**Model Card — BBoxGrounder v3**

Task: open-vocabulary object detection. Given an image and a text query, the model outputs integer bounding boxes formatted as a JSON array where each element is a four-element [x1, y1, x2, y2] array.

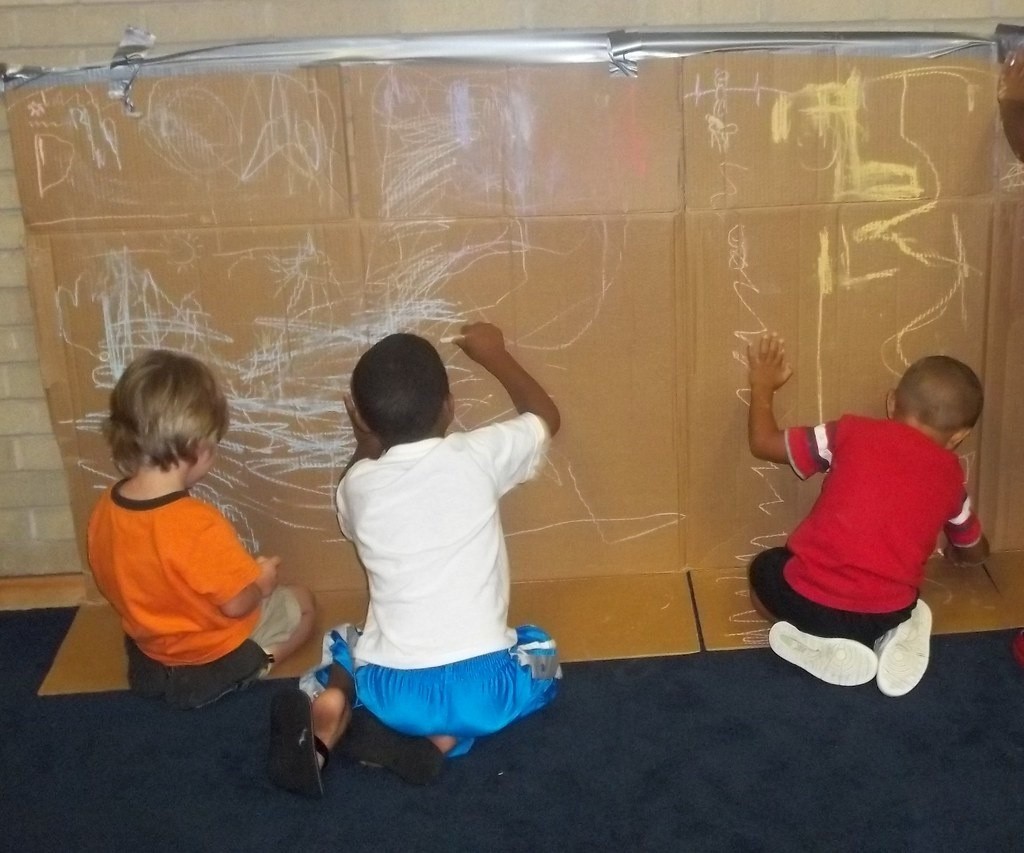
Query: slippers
[[349, 715, 445, 785], [269, 689, 326, 798]]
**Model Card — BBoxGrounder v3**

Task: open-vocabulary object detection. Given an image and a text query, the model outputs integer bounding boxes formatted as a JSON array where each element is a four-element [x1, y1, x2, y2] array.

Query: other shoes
[[872, 597, 933, 696], [123, 632, 168, 704], [768, 623, 878, 687], [169, 639, 268, 710]]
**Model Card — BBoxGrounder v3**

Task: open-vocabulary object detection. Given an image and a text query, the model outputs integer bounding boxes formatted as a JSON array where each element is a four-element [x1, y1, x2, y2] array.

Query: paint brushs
[[686, 571, 709, 654], [981, 563, 1002, 599]]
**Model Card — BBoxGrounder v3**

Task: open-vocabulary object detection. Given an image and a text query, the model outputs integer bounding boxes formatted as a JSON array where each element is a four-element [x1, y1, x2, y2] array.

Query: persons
[[264, 321, 562, 800], [86, 347, 315, 713], [746, 334, 991, 697]]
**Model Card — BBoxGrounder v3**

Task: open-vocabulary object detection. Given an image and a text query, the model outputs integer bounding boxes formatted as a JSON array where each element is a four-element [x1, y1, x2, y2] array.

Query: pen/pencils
[[440, 335, 465, 343]]
